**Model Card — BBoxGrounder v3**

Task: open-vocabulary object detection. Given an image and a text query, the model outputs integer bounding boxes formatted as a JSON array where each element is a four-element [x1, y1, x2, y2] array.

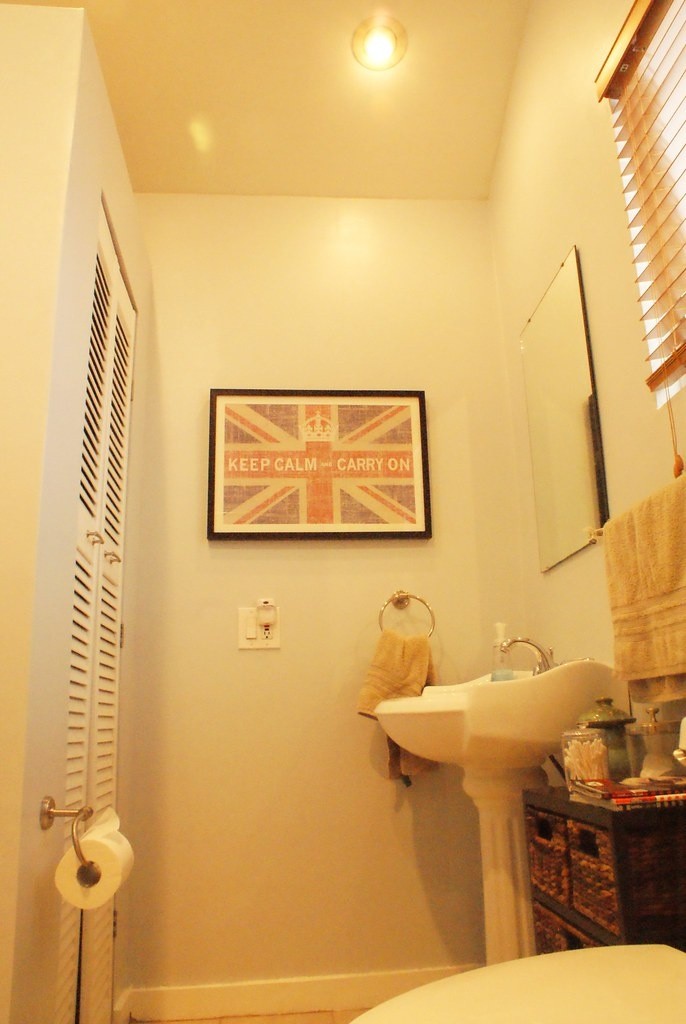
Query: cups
[[562, 696, 685, 796]]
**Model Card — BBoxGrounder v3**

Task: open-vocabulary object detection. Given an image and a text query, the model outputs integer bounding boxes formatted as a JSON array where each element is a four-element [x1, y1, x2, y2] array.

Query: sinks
[[376, 657, 616, 770]]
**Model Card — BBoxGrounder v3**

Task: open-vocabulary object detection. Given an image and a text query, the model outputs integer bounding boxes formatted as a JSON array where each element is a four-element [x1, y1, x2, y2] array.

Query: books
[[569, 777, 686, 811]]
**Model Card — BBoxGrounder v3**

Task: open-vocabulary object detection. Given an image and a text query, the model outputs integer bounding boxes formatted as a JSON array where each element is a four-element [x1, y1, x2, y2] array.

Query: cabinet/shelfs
[[522, 779, 686, 956]]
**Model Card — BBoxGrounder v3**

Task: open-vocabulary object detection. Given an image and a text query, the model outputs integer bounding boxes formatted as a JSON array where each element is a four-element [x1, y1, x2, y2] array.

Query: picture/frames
[[208, 389, 433, 539]]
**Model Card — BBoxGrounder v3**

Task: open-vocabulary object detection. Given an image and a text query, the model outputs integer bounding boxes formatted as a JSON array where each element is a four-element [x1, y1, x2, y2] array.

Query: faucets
[[500, 637, 555, 678]]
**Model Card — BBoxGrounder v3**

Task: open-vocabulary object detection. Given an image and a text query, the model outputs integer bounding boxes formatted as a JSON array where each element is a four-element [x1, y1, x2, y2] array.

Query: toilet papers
[[54, 807, 133, 912]]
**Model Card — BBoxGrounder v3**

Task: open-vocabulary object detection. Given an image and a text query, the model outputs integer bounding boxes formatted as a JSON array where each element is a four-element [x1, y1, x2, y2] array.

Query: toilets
[[349, 943, 685, 1024]]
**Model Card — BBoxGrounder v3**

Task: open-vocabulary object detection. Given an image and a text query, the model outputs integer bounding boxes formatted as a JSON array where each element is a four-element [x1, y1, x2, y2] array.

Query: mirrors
[[517, 244, 609, 573]]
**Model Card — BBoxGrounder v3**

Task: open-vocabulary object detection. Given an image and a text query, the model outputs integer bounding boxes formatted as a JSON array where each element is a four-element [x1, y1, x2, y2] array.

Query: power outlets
[[238, 607, 280, 650]]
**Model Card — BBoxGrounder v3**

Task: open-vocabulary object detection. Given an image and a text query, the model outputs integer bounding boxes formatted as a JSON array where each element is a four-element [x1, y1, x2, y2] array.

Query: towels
[[355, 629, 439, 788]]
